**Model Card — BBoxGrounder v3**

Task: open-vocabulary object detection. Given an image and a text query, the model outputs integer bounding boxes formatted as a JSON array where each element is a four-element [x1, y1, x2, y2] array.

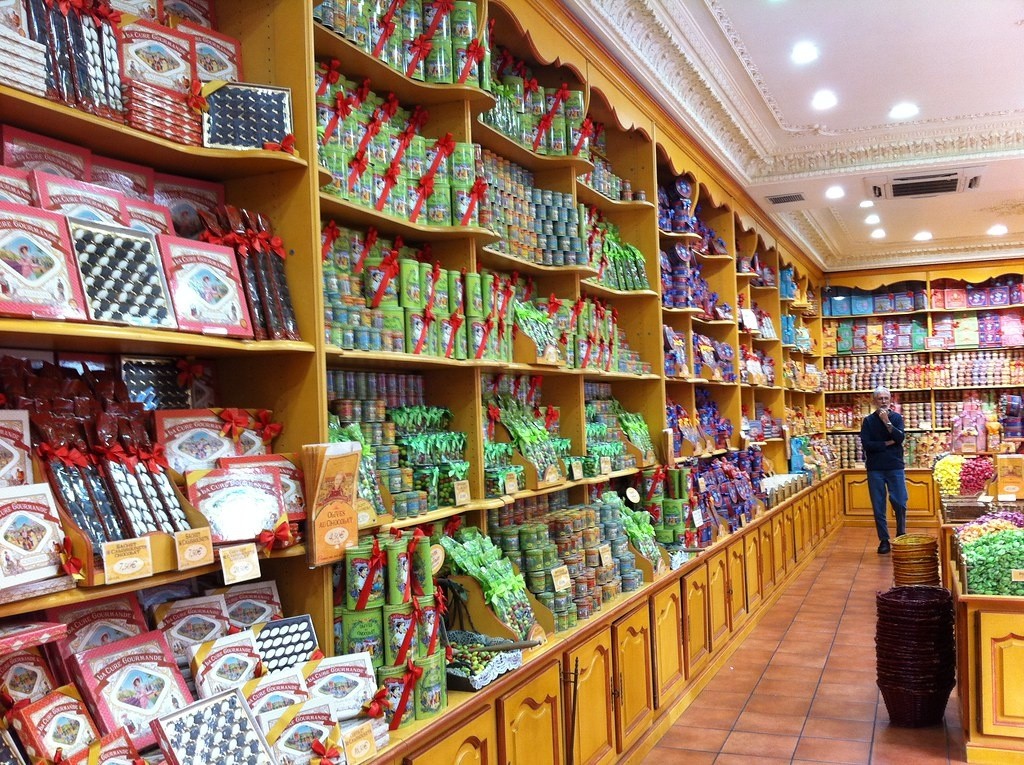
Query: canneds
[[824, 350, 1024, 469], [311, 0, 691, 727]]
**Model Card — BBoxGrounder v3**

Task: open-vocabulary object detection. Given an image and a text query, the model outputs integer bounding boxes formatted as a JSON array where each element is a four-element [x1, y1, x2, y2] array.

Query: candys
[[933, 455, 1024, 597]]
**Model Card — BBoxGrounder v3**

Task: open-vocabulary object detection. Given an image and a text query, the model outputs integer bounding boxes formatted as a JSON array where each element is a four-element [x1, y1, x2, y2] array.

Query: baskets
[[875, 585, 957, 728], [887, 533, 941, 587]]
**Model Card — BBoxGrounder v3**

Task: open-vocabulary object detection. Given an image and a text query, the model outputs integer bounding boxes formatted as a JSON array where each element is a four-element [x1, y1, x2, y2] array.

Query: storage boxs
[[1, 581, 387, 765], [1, 121, 253, 339], [820, 284, 1024, 352], [0, 0, 294, 151], [0, 344, 313, 589], [742, 400, 782, 443]]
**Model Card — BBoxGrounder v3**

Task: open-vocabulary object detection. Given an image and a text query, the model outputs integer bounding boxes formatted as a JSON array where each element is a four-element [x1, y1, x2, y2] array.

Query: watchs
[[886, 422, 892, 428]]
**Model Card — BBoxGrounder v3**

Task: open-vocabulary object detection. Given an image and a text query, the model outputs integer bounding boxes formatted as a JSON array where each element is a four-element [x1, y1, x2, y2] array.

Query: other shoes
[[878, 541, 890, 553]]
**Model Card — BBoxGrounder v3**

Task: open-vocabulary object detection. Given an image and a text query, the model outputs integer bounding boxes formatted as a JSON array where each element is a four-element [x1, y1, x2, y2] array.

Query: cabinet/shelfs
[[0, 0, 1024, 765]]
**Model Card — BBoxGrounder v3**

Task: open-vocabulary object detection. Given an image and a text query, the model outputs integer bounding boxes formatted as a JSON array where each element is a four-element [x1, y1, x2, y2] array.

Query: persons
[[861, 385, 909, 554]]
